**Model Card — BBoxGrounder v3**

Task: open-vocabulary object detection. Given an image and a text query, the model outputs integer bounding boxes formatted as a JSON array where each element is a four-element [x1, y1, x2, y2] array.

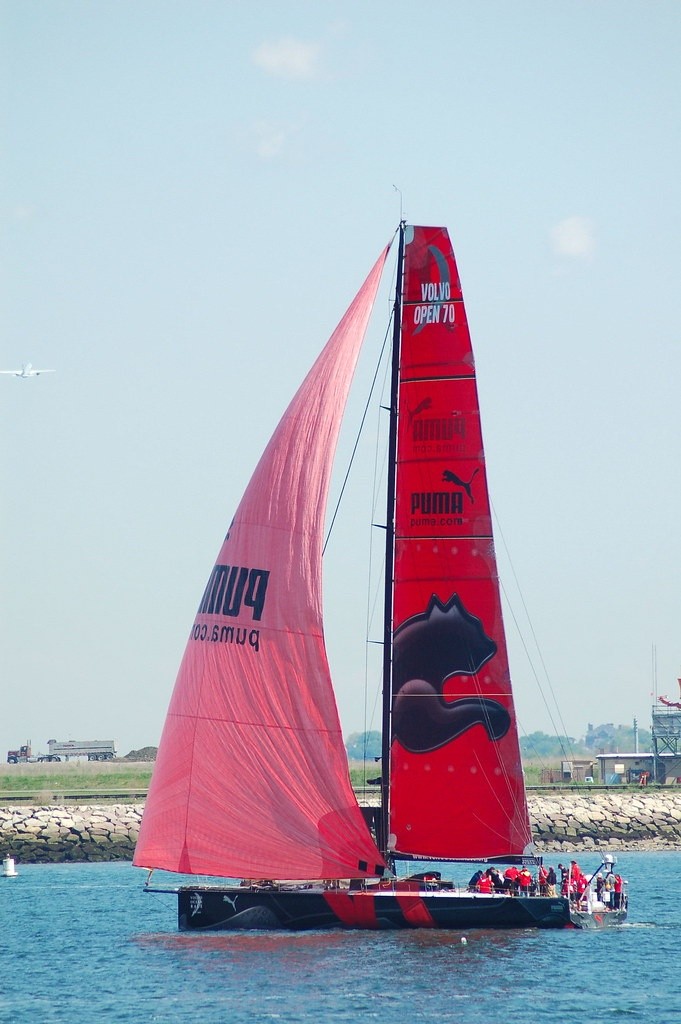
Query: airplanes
[[1, 360, 53, 380]]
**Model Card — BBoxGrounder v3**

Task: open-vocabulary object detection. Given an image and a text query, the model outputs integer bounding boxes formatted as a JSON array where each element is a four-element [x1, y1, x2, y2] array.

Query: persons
[[468, 861, 621, 910]]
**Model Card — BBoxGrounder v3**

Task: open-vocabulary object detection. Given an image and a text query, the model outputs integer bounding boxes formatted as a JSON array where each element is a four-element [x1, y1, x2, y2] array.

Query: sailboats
[[130, 184, 583, 934]]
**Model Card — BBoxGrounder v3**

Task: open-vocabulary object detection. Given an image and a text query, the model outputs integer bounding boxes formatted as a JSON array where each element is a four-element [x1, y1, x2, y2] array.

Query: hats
[[570, 860, 577, 864], [549, 866, 554, 872], [597, 873, 602, 878], [603, 869, 611, 873], [521, 868, 527, 872], [512, 866, 517, 869]]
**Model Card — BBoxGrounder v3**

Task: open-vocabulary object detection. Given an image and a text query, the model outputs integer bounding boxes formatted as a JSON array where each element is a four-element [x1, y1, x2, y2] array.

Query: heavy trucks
[[7, 739, 117, 764]]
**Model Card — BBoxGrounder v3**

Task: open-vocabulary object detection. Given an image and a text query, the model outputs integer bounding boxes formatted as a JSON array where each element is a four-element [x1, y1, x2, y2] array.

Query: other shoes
[[577, 906, 581, 910], [606, 909, 611, 912]]
